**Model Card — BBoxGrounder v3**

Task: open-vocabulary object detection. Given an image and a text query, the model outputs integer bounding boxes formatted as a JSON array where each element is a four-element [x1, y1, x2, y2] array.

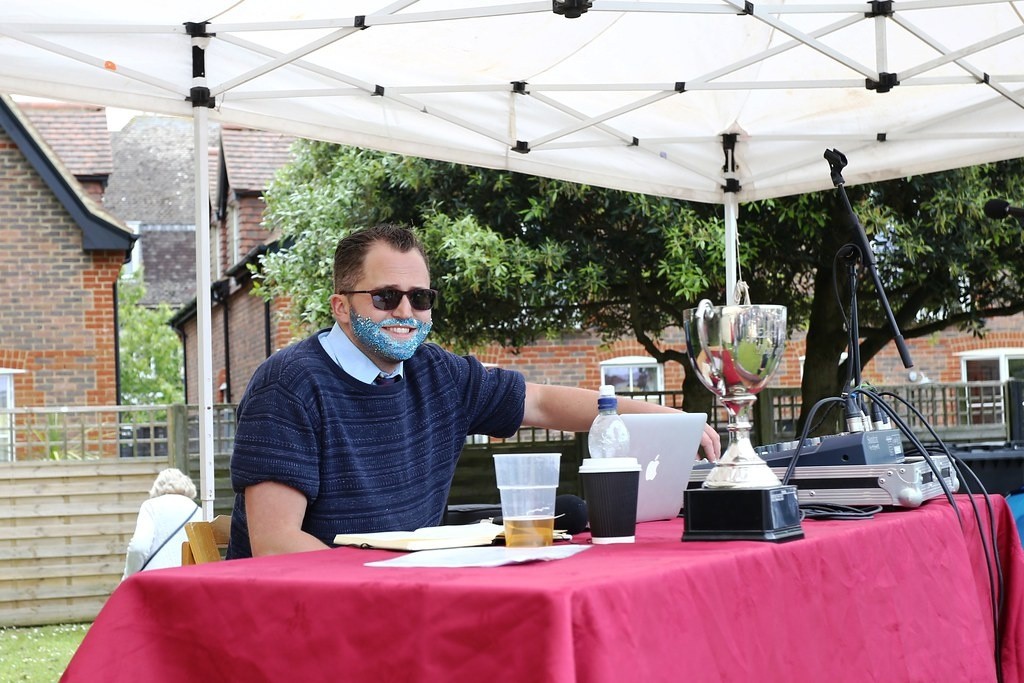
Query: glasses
[[340, 288, 438, 310]]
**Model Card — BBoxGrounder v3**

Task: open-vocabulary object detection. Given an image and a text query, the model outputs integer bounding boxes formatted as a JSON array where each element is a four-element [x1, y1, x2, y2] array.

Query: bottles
[[588, 385, 630, 458]]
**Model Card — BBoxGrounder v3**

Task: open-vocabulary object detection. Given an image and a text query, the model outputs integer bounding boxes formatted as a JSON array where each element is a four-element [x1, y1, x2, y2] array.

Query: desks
[[58, 493, 1024, 683]]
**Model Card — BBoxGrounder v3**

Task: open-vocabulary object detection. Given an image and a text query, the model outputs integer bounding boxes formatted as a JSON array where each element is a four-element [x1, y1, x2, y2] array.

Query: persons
[[224, 225, 721, 561], [121, 469, 203, 582]]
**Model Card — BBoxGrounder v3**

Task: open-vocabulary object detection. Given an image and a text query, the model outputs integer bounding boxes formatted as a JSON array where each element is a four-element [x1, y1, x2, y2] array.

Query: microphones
[[470, 496, 590, 536]]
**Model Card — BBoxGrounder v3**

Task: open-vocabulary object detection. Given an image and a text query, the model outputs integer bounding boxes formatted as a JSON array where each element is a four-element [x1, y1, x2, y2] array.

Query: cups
[[493, 453, 562, 548], [579, 458, 643, 545]]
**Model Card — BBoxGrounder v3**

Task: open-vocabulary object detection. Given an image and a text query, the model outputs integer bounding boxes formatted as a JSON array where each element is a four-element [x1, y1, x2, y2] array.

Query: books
[[334, 523, 571, 551]]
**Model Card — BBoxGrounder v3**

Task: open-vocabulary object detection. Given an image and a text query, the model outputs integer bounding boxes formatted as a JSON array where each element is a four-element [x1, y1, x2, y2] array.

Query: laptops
[[619, 411, 708, 523]]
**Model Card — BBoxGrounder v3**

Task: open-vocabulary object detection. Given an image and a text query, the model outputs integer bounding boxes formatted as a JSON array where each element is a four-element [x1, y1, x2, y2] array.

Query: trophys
[[682, 299, 804, 542]]
